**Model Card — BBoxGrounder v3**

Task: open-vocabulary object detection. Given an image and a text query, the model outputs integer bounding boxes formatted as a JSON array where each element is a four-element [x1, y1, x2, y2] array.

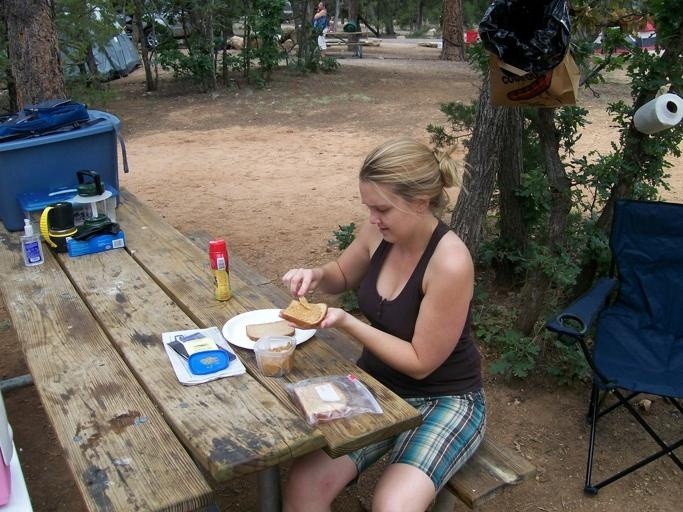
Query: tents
[[592, 15, 663, 54], [55, 3, 144, 82]]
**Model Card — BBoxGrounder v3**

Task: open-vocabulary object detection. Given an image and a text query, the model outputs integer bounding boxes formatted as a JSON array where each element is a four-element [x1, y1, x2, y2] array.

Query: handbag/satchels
[[0, 99, 90, 142], [478, 0, 580, 107]]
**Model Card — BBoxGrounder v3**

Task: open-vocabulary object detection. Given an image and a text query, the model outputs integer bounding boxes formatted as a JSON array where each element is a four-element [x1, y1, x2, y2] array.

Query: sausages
[[507, 72, 552, 101]]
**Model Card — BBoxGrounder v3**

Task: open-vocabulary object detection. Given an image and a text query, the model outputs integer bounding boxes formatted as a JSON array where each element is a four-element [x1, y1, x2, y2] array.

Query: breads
[[283, 299, 327, 328], [246, 319, 295, 340], [292, 382, 348, 424]]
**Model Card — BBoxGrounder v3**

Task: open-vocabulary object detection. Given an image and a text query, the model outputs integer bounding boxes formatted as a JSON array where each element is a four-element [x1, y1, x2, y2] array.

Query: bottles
[[208, 239, 232, 301], [21, 218, 44, 267]]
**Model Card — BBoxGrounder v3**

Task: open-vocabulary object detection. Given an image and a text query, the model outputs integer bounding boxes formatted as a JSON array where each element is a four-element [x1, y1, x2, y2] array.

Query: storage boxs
[[0, 110, 120, 231]]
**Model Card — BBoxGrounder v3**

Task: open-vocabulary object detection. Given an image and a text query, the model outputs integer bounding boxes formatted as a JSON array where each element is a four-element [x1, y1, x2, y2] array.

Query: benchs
[[327, 43, 369, 58], [173, 224, 535, 512]]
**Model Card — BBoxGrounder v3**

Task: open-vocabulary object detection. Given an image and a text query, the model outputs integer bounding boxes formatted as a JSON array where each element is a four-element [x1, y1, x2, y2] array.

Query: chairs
[[544, 197, 683, 495]]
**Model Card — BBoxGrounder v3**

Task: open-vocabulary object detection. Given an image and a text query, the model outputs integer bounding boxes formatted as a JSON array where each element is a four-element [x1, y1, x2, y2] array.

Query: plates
[[221, 309, 318, 351]]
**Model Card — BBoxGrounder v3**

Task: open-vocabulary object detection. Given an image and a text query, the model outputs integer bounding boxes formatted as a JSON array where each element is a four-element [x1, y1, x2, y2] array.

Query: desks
[[0, 197, 420, 512], [324, 32, 367, 58]]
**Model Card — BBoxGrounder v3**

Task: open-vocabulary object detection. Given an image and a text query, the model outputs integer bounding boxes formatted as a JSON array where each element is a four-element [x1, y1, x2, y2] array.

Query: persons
[[279, 139, 486, 512], [312, 1, 330, 57]]
